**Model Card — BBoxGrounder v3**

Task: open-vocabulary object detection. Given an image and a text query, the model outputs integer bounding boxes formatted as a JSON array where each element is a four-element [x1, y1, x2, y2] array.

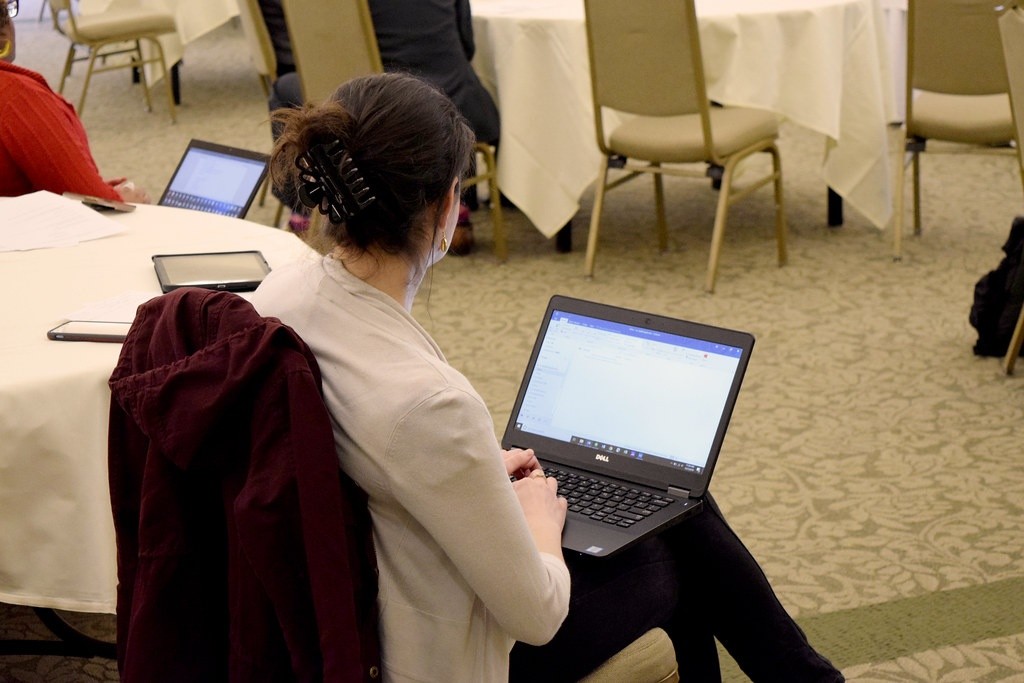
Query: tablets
[[151, 250, 273, 295]]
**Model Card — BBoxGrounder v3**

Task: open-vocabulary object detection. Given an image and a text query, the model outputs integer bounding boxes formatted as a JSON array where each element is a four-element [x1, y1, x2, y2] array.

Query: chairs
[[281, 0, 508, 265], [108, 287, 680, 683], [584, 0, 789, 295], [48, 0, 178, 127], [237, 0, 278, 101], [893, 0, 1024, 261]]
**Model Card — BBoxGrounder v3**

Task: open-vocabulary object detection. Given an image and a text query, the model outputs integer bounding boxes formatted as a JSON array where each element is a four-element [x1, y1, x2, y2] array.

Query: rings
[[534, 474, 549, 484]]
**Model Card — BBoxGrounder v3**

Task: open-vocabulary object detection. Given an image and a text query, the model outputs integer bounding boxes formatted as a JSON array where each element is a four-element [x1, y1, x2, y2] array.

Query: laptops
[[156, 139, 272, 219], [500, 295, 756, 562]]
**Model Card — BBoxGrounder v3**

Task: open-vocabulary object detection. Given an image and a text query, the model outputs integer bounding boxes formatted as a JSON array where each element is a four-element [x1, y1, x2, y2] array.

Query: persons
[[249, 70, 844, 683], [0, 1, 154, 206], [261, 0, 297, 83], [269, 2, 501, 257]]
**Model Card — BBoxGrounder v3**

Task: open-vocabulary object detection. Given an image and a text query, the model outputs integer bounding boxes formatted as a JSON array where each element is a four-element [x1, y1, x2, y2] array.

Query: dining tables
[[78, 0, 240, 107], [0, 196, 330, 661], [470, 1, 909, 256]]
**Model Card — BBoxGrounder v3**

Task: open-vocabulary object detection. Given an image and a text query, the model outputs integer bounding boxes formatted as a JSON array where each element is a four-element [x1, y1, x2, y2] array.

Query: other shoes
[[446, 225, 476, 257]]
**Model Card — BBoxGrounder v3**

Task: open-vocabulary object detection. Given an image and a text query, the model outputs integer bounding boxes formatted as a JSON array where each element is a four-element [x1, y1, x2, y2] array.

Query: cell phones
[[62, 191, 136, 212], [47, 319, 133, 343]]
